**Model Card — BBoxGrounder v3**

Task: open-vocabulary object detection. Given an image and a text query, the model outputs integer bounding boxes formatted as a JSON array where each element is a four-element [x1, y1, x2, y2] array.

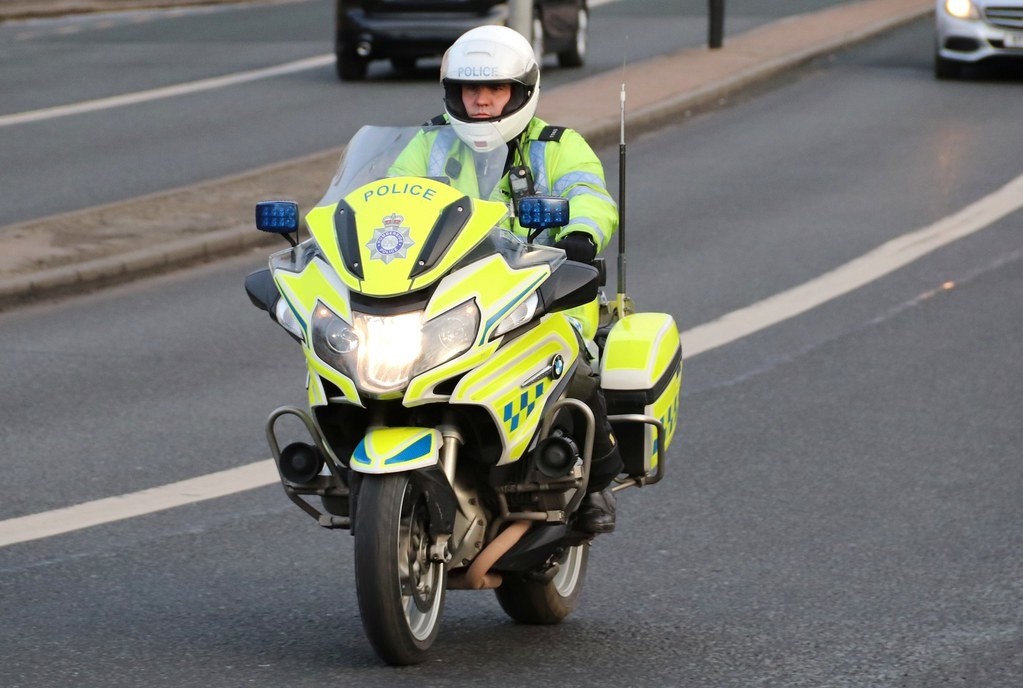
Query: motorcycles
[[242, 125, 687, 667]]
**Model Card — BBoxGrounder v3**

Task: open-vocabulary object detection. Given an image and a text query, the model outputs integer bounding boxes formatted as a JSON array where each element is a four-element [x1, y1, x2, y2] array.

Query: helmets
[[440, 25, 539, 153]]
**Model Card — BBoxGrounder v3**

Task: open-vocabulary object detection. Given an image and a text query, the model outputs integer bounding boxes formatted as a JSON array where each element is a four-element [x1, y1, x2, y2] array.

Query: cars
[[333, 1, 592, 80], [933, 1, 1023, 80]]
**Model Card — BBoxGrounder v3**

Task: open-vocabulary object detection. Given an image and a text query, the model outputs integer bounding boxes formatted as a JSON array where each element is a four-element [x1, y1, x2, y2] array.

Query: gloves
[[552, 231, 597, 264], [290, 242, 319, 265]]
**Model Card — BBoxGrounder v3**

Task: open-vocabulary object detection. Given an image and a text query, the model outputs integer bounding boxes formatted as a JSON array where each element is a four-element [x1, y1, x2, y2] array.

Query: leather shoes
[[579, 484, 618, 533]]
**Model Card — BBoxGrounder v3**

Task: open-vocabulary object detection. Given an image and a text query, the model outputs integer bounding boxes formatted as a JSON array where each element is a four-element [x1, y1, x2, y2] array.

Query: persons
[[383, 24, 625, 533]]
